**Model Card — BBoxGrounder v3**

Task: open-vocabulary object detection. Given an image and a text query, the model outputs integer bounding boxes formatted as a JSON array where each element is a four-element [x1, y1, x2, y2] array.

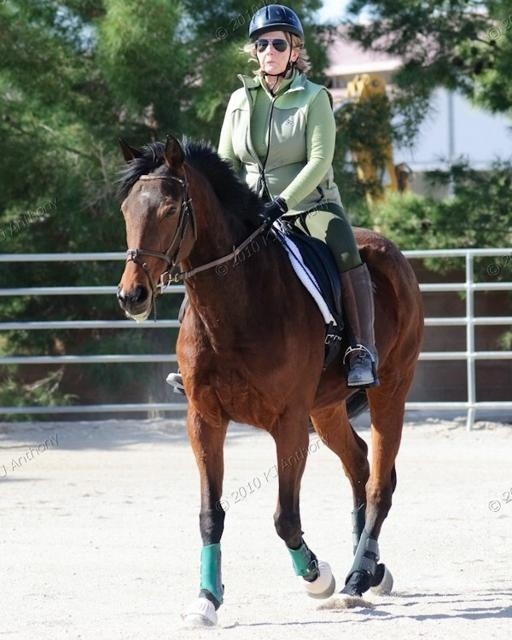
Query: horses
[[113, 131, 426, 627]]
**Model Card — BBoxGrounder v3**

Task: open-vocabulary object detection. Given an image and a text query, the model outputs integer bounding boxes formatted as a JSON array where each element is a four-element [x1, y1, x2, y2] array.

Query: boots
[[166, 371, 185, 391], [334, 259, 379, 388]]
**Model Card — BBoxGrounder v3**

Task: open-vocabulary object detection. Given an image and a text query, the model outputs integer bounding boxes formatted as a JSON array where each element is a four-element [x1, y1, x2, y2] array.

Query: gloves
[[256, 197, 291, 232]]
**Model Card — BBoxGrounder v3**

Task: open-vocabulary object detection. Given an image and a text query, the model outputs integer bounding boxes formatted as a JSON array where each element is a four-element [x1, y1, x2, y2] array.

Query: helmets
[[246, 4, 305, 39]]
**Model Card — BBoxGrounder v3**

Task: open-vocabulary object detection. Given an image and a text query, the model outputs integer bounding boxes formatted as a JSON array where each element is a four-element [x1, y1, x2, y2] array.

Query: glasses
[[254, 39, 289, 53]]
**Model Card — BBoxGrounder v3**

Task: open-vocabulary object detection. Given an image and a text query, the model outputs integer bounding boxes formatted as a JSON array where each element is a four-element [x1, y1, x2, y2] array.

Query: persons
[[164, 3, 380, 392]]
